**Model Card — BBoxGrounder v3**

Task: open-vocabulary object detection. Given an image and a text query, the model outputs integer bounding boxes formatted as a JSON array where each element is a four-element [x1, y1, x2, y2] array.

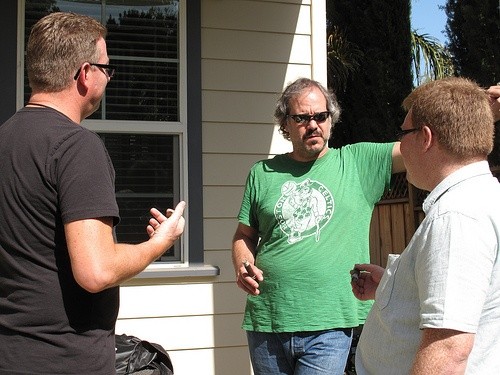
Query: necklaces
[[27, 101, 72, 120]]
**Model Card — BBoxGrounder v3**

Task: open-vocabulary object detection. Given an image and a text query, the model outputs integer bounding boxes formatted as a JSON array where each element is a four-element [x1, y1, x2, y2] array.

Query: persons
[[348, 75, 500, 375], [0, 10, 186, 375], [231, 77, 500, 375]]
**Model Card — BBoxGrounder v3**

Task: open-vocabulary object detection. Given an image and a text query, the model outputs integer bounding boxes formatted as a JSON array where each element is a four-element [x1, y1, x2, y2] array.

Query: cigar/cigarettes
[[352, 264, 360, 282], [243, 260, 259, 284]]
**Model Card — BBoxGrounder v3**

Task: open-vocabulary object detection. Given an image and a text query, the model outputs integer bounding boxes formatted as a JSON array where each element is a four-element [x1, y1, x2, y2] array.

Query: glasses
[[394, 127, 434, 142], [74, 64, 116, 80], [285, 111, 330, 123]]
